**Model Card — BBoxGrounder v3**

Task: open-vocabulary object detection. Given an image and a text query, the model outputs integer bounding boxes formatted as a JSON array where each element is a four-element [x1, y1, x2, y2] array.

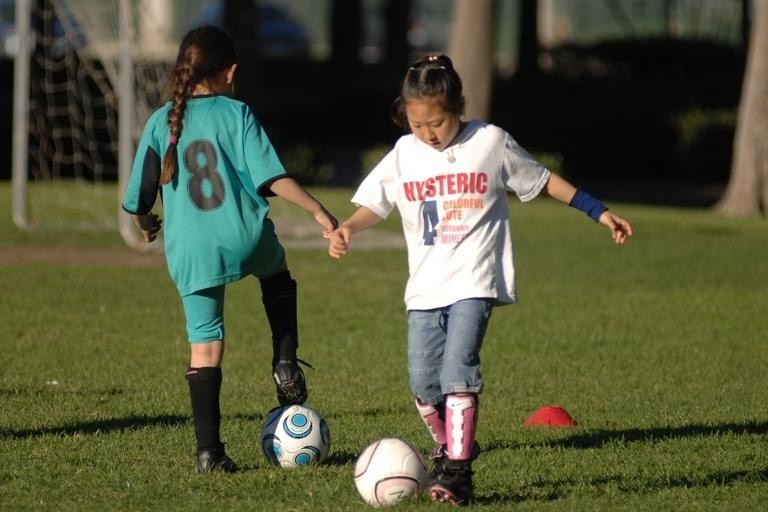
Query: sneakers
[[195, 448, 235, 471], [274, 357, 315, 403], [427, 438, 481, 506]]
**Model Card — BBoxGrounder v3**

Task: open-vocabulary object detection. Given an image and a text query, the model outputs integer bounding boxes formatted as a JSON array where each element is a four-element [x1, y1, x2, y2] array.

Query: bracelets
[[567, 187, 610, 224]]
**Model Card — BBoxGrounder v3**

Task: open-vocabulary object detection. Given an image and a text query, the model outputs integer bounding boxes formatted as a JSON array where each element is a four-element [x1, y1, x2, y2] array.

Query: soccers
[[260, 404, 330, 466], [354, 437, 429, 509]]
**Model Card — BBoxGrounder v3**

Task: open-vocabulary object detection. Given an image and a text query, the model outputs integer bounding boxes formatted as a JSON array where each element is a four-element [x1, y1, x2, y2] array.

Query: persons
[[121, 25, 339, 475], [327, 54, 634, 508]]
